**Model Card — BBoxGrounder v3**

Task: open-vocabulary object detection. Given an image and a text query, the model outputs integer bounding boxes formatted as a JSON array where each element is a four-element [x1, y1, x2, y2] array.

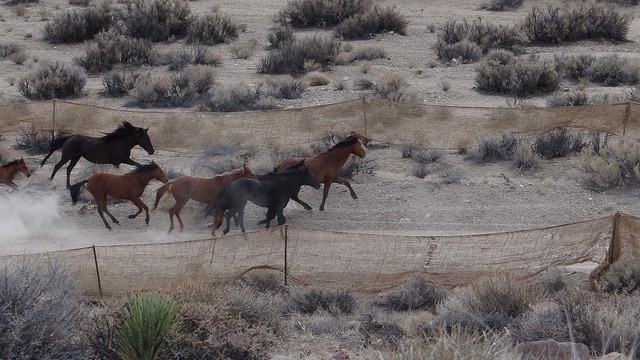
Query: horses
[[41, 120, 154, 189], [151, 162, 256, 237], [201, 165, 321, 235], [0, 158, 30, 194], [232, 159, 305, 227], [274, 131, 366, 211], [70, 159, 168, 231]]
